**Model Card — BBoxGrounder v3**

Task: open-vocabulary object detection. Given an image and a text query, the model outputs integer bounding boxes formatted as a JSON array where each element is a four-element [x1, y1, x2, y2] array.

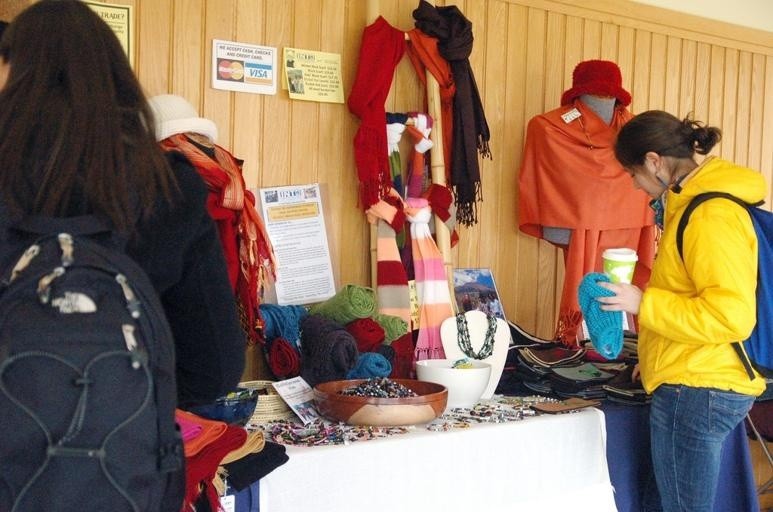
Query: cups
[[602, 248, 639, 284]]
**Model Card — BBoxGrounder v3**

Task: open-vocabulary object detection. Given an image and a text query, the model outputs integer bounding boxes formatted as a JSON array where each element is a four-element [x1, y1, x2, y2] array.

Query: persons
[[1, 0, 187, 512], [519, 59, 658, 347], [593, 110, 766, 512], [145, 94, 277, 352]]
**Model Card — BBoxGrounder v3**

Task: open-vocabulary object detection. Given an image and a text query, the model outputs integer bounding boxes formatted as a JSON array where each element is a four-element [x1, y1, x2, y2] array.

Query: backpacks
[[677, 192, 773, 379], [0, 212, 185, 512]]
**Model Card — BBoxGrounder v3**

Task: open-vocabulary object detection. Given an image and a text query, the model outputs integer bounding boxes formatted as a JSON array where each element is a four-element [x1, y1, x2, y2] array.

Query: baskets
[[238, 380, 295, 423]]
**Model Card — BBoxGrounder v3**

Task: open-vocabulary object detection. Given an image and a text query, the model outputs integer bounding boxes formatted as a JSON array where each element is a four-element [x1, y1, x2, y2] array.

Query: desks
[[195, 365, 759, 512]]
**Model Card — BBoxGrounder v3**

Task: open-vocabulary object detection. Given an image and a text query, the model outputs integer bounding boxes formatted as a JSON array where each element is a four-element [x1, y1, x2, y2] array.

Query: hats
[[561, 61, 631, 106], [578, 272, 623, 359], [138, 94, 219, 143]]
